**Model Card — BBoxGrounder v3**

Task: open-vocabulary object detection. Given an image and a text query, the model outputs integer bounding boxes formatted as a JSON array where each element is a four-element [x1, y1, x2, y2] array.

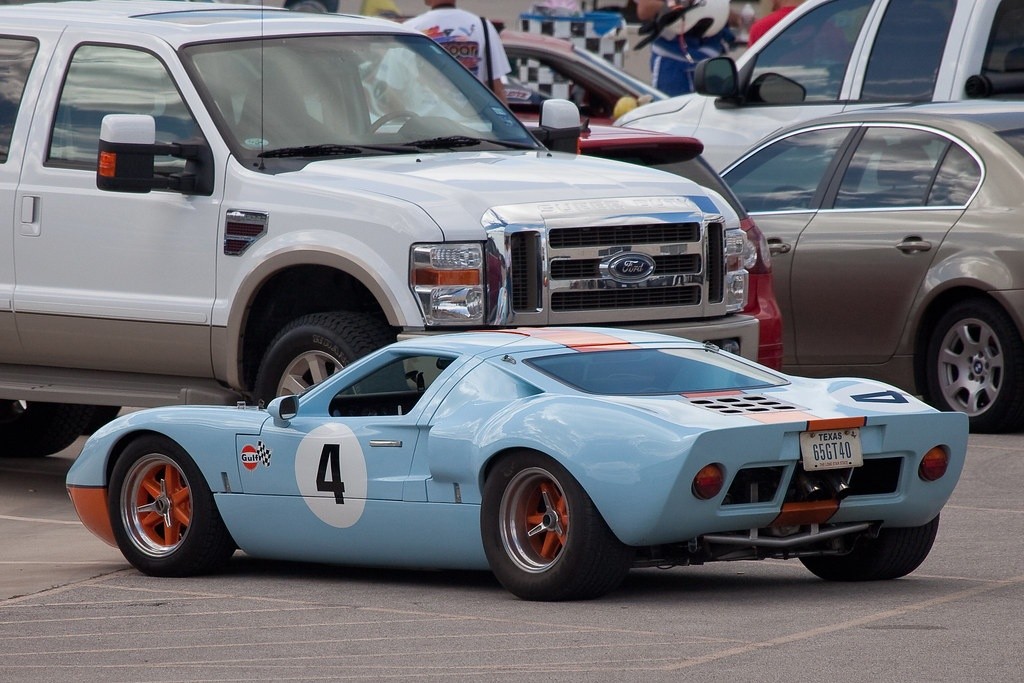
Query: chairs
[[873, 4, 946, 79], [74, 67, 177, 152], [238, 78, 333, 147], [873, 144, 932, 205]]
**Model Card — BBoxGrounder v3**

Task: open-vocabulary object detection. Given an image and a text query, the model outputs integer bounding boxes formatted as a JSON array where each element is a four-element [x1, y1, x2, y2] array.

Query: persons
[[376, 0, 512, 131], [634, 0, 847, 97]]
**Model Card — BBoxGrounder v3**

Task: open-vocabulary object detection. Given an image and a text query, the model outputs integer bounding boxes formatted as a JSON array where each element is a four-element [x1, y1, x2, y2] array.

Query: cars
[[715, 109, 1024, 433]]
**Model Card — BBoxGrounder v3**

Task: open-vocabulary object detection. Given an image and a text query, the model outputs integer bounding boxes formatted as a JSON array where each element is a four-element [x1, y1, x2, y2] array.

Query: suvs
[[0, 0, 762, 457], [359, 0, 1024, 375]]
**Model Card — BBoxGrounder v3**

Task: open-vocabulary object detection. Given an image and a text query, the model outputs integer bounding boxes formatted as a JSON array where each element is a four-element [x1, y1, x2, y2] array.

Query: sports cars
[[63, 322, 972, 600]]
